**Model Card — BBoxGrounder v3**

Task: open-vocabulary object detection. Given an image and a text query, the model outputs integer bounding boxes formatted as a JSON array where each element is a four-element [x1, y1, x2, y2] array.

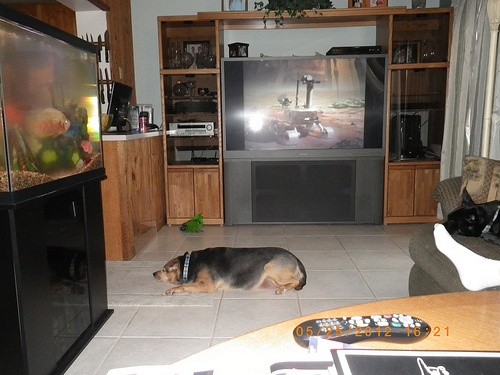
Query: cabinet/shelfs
[[165, 166, 224, 228], [156, 6, 455, 166], [385, 163, 438, 225]]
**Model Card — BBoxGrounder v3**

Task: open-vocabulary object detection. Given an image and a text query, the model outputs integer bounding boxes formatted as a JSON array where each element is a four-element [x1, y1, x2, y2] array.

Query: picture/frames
[[348, 0, 388, 8], [330, 348, 500, 375], [222, 0, 249, 11], [392, 40, 420, 63], [183, 40, 210, 69]]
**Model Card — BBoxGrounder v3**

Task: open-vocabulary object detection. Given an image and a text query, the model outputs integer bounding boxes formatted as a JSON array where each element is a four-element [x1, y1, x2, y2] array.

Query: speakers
[[391, 114, 422, 159]]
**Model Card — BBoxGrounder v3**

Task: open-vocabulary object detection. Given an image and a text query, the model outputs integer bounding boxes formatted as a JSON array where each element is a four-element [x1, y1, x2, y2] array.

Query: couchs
[[408, 176, 499, 297]]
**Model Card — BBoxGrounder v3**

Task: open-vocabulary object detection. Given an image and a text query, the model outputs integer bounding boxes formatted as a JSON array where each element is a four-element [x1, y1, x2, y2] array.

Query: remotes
[[293, 312, 432, 349]]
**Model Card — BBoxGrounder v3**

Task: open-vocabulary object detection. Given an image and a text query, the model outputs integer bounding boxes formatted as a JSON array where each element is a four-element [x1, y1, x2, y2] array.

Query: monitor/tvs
[[221, 54, 388, 159], [107, 81, 132, 134]]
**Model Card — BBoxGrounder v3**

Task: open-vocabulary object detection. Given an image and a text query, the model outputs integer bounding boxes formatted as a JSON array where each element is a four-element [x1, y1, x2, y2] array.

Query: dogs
[[153, 247, 306, 295], [433, 187, 500, 237]]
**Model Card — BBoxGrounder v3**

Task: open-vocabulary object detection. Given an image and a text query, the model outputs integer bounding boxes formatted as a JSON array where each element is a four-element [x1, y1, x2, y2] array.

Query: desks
[[170, 291, 500, 375], [101, 128, 165, 263]]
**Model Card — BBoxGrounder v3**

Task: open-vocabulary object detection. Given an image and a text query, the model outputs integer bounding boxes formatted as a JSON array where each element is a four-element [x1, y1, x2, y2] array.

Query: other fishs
[[24, 108, 71, 139]]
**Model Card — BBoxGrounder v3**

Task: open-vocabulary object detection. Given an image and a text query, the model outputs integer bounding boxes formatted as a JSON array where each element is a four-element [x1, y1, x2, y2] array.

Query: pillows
[[458, 152, 500, 204]]
